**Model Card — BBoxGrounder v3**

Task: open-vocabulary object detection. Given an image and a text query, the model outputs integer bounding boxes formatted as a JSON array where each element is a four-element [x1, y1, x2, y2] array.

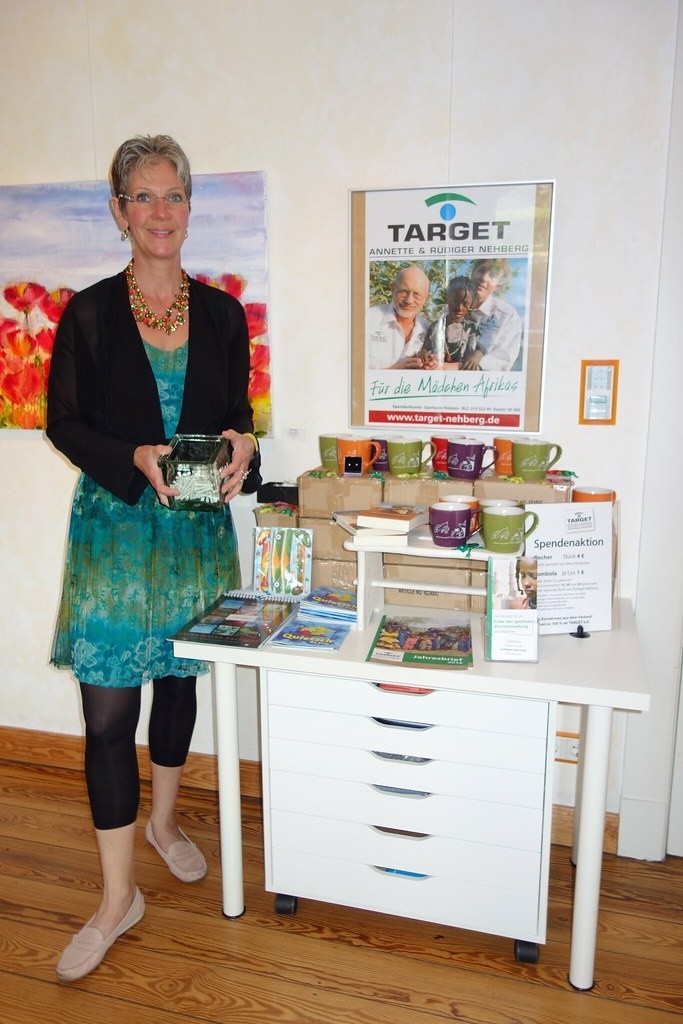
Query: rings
[[240, 469, 252, 481]]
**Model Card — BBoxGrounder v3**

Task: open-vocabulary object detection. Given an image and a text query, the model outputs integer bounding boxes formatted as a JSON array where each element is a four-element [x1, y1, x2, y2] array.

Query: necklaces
[[125, 261, 190, 334]]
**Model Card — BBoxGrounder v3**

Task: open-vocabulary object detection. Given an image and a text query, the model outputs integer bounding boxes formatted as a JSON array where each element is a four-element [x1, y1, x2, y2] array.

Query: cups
[[430, 435, 465, 473], [319, 434, 353, 471], [512, 439, 562, 480], [370, 434, 401, 470], [478, 498, 525, 539], [440, 493, 479, 532], [337, 436, 382, 476], [483, 507, 537, 551], [446, 439, 499, 480], [572, 486, 616, 509], [429, 502, 483, 547], [387, 437, 436, 476], [494, 436, 528, 475]]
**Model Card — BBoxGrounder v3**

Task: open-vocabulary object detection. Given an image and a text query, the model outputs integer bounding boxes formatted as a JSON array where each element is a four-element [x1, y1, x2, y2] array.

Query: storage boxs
[[158, 433, 234, 509], [256, 462, 572, 611]]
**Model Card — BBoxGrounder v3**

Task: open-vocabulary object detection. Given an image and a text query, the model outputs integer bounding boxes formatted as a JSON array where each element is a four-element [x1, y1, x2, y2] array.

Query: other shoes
[[56, 887, 146, 982], [146, 819, 208, 882]]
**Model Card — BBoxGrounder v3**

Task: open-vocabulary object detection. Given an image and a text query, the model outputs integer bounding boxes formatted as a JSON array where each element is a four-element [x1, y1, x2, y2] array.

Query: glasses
[[118, 193, 190, 208]]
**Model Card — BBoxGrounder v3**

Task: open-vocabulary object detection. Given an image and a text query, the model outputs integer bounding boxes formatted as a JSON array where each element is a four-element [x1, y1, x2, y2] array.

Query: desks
[[173, 599, 653, 991]]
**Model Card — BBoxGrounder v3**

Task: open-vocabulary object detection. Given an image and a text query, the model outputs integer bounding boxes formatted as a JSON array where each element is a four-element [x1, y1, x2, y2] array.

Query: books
[[166, 528, 358, 652]]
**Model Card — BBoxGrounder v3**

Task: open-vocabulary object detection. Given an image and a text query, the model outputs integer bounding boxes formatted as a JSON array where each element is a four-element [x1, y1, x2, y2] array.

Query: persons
[[419, 277, 479, 370], [506, 559, 537, 610], [380, 620, 472, 653], [425, 259, 523, 371], [45, 138, 259, 980], [369, 268, 437, 370]]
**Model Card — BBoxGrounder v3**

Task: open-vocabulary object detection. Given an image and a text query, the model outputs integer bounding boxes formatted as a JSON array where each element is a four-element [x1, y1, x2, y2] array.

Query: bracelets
[[242, 433, 258, 456]]
